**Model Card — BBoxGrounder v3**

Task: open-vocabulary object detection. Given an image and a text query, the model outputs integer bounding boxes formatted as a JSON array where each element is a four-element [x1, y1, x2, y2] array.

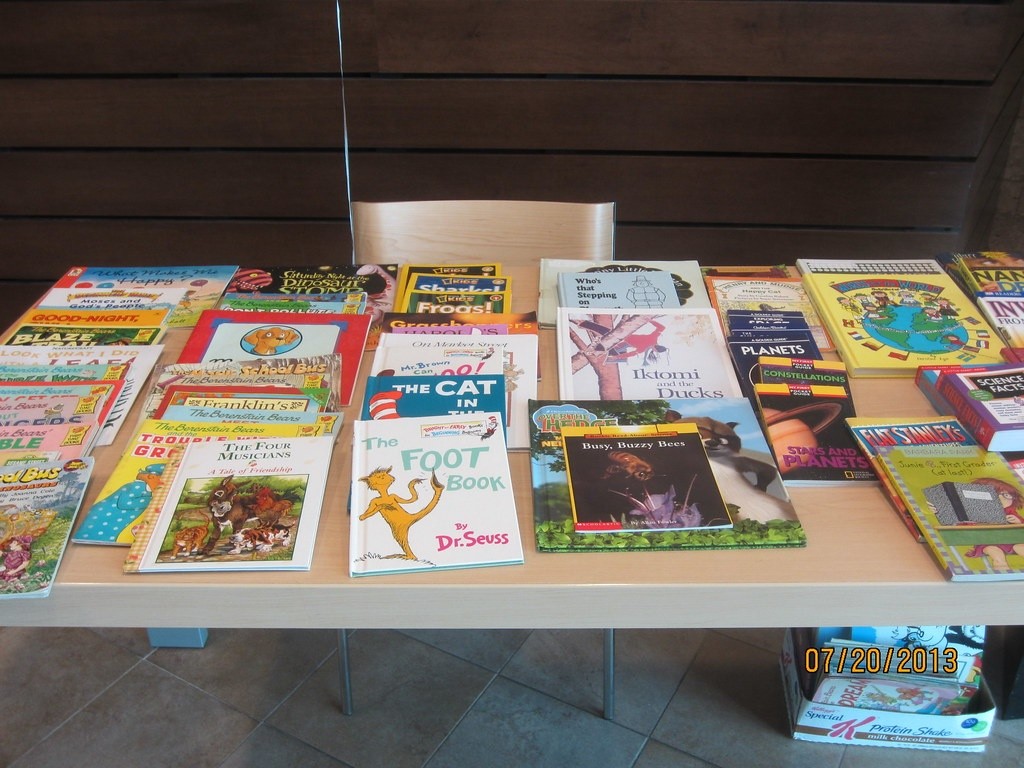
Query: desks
[[0, 265, 1024, 629]]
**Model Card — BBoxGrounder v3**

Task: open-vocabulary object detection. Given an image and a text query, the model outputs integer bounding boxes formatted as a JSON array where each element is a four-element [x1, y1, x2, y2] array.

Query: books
[[528, 396, 807, 552], [376, 260, 542, 381], [345, 373, 508, 514], [0, 262, 398, 600], [555, 269, 681, 309], [555, 305, 743, 399], [561, 422, 733, 534], [353, 331, 541, 452], [790, 624, 987, 718], [700, 252, 1023, 582], [351, 410, 524, 578], [536, 257, 712, 331]]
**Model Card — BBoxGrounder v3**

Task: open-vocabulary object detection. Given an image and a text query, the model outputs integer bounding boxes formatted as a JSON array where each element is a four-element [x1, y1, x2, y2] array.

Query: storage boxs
[[778, 625, 997, 753]]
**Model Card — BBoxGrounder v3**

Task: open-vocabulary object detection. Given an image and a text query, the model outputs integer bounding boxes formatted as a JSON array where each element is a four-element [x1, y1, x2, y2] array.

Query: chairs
[[336, 200, 618, 720]]
